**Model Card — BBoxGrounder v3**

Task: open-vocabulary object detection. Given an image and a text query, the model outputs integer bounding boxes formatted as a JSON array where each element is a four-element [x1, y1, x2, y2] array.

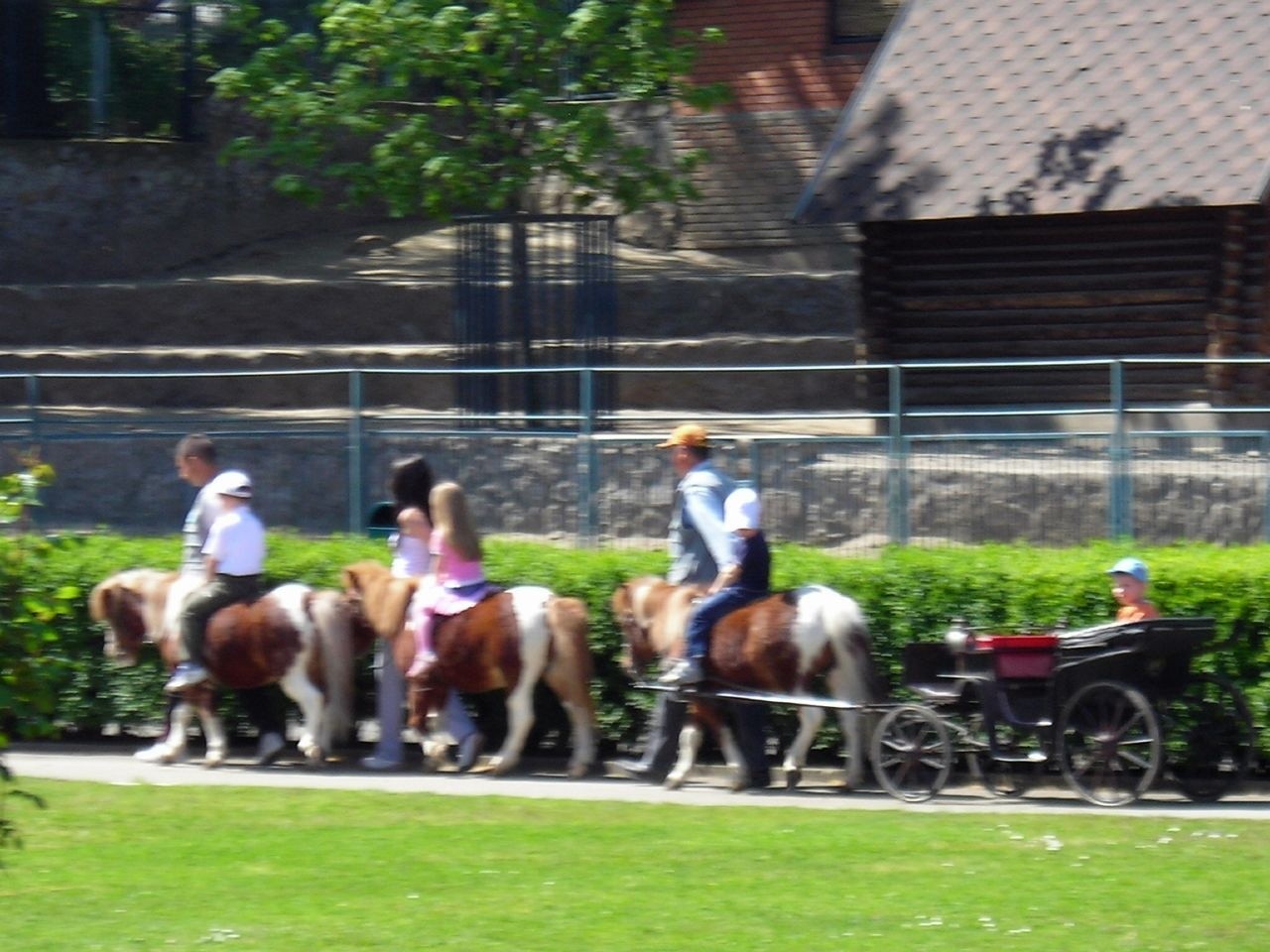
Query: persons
[[361, 454, 486, 774], [1104, 556, 1158, 623], [624, 424, 772, 792], [132, 432, 286, 763]]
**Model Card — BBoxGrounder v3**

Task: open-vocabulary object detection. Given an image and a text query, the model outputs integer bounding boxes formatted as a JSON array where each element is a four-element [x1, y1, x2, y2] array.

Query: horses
[[610, 573, 884, 796], [340, 560, 602, 779], [88, 569, 378, 771]]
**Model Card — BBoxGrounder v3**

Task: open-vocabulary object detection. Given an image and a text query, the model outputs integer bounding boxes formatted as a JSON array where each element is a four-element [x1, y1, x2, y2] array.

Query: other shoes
[[659, 662, 705, 686], [404, 655, 439, 679], [359, 756, 403, 770], [163, 661, 207, 691], [456, 733, 485, 771], [749, 768, 771, 787], [255, 729, 286, 763], [622, 764, 667, 783]]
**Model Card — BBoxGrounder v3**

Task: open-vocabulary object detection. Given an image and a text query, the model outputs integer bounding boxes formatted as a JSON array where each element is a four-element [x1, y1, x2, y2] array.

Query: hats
[[211, 471, 252, 498], [1101, 557, 1149, 583], [654, 425, 707, 449], [722, 489, 759, 533]]
